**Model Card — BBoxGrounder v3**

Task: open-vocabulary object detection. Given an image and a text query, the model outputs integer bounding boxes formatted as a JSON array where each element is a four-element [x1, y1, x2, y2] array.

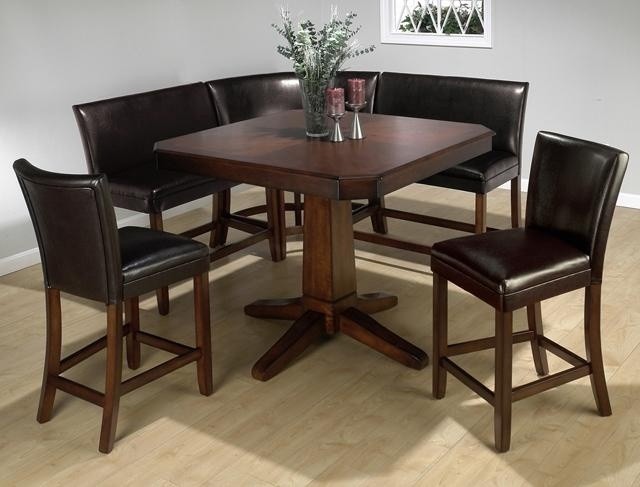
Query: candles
[[347, 78, 365, 105], [325, 86, 345, 114]]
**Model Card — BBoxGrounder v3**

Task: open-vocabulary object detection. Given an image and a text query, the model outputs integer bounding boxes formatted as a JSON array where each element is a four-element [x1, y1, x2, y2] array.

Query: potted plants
[[271, 2, 375, 137]]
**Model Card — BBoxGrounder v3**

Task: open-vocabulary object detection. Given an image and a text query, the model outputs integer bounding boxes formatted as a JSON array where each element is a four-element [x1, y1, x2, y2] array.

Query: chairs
[[72, 81, 281, 316], [431, 130, 628, 453], [205, 71, 380, 260], [13, 158, 214, 453], [352, 72, 529, 258]]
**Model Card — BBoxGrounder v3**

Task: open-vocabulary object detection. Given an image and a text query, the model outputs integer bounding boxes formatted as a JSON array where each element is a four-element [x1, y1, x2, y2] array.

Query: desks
[[155, 109, 497, 382]]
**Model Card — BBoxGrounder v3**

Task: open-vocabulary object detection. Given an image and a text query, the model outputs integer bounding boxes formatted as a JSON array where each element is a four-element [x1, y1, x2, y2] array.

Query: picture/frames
[[379, 0, 492, 48]]
